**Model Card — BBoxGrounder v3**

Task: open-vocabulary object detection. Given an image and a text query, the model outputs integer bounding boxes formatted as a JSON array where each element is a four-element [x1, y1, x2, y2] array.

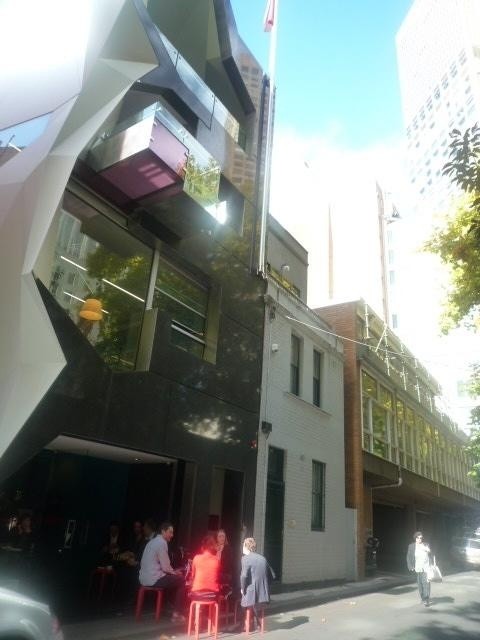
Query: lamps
[[79, 299, 103, 321]]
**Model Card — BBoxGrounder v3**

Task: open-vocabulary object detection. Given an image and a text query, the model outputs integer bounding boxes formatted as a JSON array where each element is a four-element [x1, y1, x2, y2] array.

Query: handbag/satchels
[[426, 555, 445, 585]]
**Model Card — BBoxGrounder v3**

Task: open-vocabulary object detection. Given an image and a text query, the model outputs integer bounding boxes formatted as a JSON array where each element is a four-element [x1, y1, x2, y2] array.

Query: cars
[[0, 589, 67, 640]]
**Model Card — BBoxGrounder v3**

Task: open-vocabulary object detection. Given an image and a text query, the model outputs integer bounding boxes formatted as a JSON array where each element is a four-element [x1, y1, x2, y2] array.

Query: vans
[[449, 530, 479, 568]]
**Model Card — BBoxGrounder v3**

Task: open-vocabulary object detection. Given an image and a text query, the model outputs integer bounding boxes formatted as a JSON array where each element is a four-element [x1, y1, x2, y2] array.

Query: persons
[[214, 528, 234, 600], [128, 518, 159, 589], [138, 522, 187, 623], [96, 520, 128, 553], [239, 537, 276, 631], [185, 534, 223, 602], [405, 530, 436, 606], [8, 513, 40, 556], [130, 519, 144, 544]]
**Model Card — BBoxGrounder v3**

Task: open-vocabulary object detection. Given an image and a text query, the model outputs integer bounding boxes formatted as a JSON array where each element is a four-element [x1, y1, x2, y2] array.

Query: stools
[[88, 569, 265, 640]]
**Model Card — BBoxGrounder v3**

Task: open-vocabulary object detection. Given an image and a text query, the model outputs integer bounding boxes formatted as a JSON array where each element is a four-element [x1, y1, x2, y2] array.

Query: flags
[[263, 0, 274, 33]]
